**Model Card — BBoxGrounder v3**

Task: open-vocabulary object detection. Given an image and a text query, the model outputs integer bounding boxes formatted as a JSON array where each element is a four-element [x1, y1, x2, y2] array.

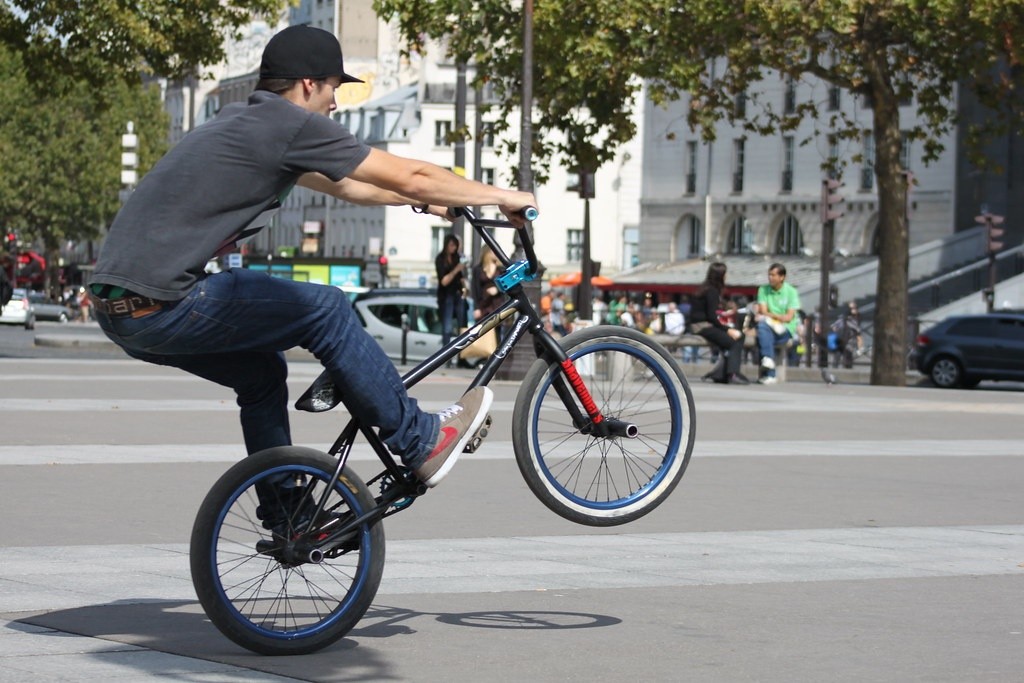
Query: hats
[[260, 24, 365, 83]]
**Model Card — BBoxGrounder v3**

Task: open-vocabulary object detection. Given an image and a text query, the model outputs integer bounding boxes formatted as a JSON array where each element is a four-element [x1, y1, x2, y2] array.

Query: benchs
[[649, 333, 793, 383]]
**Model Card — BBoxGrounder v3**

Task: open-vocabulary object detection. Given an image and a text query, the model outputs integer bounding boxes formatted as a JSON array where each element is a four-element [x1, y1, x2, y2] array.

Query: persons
[[540, 283, 866, 368], [91, 25, 540, 553], [689, 263, 750, 385], [753, 263, 801, 384], [433, 233, 474, 370], [78, 287, 93, 323], [471, 243, 507, 352]]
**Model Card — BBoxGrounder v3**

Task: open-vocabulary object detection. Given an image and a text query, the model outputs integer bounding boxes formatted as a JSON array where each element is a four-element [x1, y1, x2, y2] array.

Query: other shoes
[[758, 356, 777, 385]]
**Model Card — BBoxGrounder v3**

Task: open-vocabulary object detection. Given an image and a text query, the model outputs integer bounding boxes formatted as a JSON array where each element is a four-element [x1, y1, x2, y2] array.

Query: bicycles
[[185, 195, 697, 661]]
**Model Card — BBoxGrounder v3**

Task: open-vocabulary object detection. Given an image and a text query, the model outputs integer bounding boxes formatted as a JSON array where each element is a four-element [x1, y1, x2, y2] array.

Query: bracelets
[[411, 203, 429, 213]]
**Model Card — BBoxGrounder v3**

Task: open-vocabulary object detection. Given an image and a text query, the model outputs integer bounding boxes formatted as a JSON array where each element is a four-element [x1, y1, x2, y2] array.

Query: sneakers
[[415, 386, 494, 489], [277, 513, 360, 562]]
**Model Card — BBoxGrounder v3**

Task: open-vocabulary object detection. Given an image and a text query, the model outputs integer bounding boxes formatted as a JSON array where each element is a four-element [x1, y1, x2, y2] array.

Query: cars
[[26, 290, 76, 331], [348, 286, 494, 370], [912, 308, 1024, 394], [0, 289, 37, 331]]
